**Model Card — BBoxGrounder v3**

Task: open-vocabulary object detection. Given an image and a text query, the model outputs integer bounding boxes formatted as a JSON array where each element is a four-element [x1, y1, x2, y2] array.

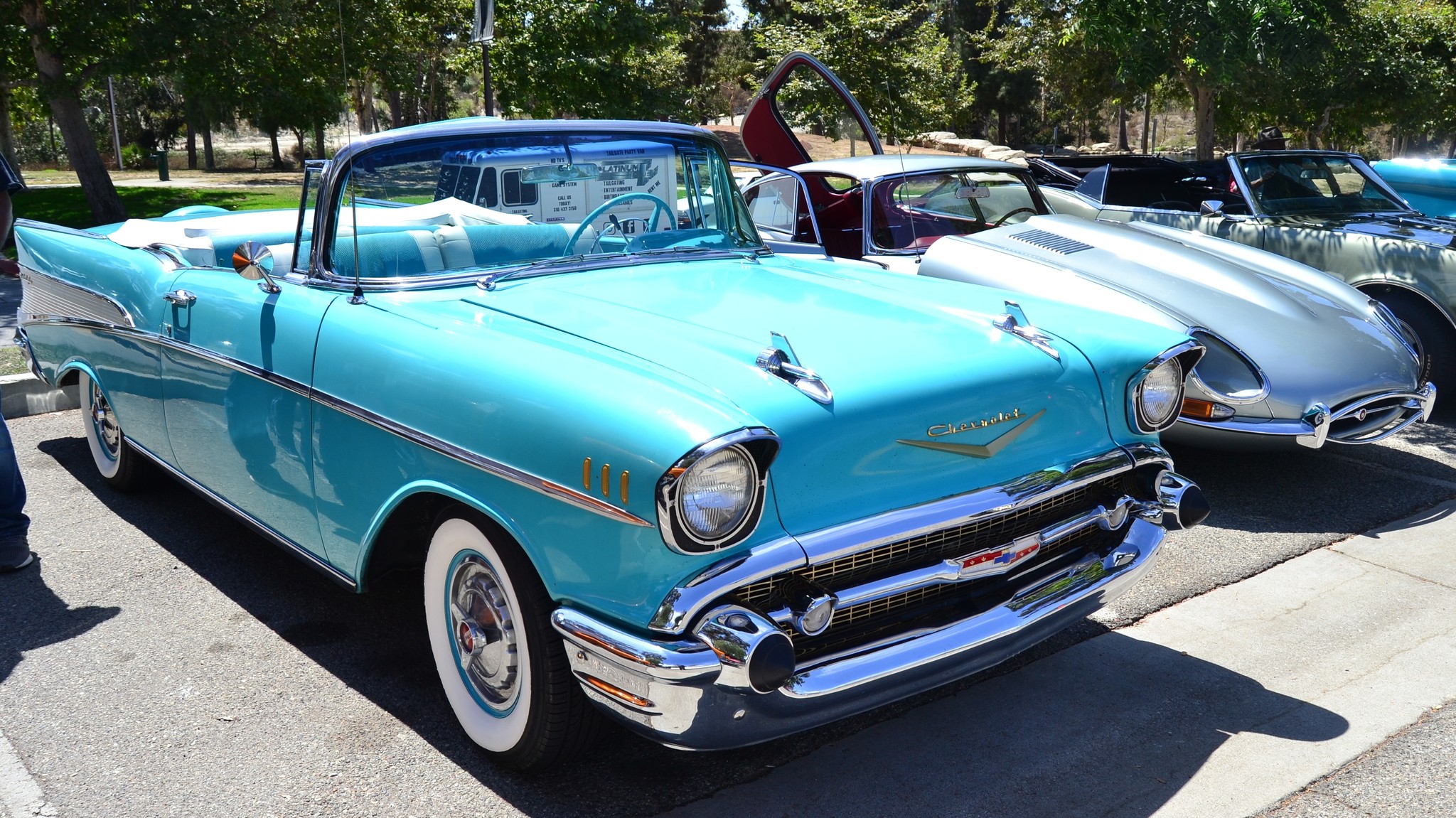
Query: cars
[[911, 146, 1456, 394]]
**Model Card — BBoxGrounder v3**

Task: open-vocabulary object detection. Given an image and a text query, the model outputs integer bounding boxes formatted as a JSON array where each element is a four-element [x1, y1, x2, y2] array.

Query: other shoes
[[0, 534, 34, 571]]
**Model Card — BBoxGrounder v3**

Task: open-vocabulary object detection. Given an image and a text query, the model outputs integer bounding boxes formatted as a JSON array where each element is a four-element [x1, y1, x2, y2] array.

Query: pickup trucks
[[17, 116, 1212, 797], [739, 52, 1437, 453]]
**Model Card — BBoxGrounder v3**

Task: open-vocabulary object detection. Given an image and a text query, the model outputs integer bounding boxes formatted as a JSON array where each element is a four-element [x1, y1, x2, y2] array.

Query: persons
[[0, 413, 33, 570], [1233, 123, 1324, 201], [227, 370, 302, 498]]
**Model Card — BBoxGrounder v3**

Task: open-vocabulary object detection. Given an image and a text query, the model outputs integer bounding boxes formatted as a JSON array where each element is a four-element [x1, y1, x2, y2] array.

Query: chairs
[[266, 229, 444, 277], [435, 221, 603, 268]]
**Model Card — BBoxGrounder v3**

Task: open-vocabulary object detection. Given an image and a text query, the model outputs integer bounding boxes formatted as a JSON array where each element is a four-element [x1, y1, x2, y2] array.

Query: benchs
[[1104, 182, 1250, 216], [178, 220, 438, 267], [800, 221, 958, 260]]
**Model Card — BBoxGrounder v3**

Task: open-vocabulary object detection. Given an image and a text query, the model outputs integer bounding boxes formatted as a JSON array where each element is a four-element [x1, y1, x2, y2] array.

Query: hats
[[1250, 126, 1292, 149]]
